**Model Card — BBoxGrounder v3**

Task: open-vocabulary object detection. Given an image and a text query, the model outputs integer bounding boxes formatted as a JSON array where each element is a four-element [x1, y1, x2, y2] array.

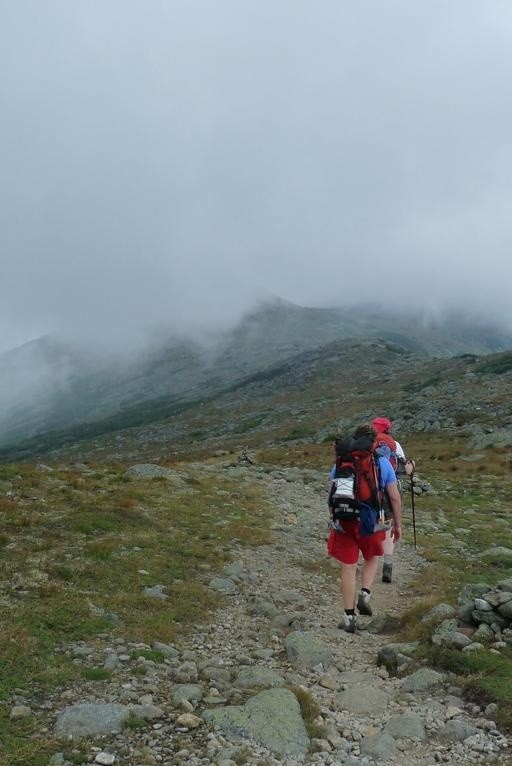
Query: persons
[[370, 416, 418, 583], [327, 425, 402, 634]]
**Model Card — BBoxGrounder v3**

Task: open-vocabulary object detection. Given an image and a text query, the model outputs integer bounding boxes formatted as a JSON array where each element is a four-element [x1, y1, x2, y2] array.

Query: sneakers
[[382, 564, 392, 584], [356, 591, 372, 617], [336, 610, 357, 634]]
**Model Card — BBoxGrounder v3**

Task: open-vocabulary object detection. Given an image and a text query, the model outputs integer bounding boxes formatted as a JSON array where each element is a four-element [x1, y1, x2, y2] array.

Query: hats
[[369, 416, 394, 434], [351, 424, 376, 442]]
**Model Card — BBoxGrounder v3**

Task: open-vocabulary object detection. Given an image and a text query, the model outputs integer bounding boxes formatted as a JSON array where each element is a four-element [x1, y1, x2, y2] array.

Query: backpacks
[[372, 433, 399, 474], [326, 432, 391, 535]]
